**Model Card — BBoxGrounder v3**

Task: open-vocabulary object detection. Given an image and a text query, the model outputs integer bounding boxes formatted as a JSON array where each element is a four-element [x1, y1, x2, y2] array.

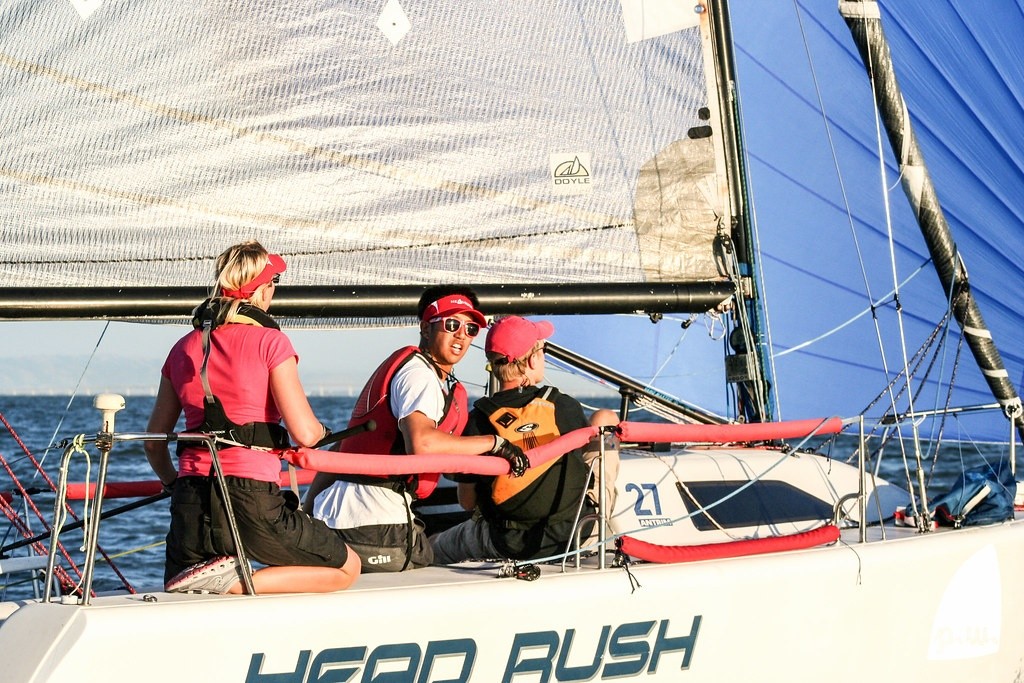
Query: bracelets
[[160, 478, 181, 488]]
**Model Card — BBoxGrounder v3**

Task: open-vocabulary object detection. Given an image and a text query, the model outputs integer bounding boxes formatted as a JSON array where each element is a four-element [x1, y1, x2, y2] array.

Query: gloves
[[491, 434, 531, 478], [320, 420, 333, 440]]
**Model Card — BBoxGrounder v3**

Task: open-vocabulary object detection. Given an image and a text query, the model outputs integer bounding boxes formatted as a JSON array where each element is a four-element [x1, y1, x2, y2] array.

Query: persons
[[297, 284, 530, 572], [429, 315, 620, 565], [143, 240, 362, 593]]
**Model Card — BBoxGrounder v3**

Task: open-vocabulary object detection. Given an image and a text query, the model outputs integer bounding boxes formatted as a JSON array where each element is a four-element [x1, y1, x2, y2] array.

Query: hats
[[213, 254, 287, 300], [421, 293, 488, 341], [483, 315, 553, 365]]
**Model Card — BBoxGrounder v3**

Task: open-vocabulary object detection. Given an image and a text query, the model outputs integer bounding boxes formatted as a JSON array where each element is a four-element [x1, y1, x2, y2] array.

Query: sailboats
[[0, 0, 1023, 683]]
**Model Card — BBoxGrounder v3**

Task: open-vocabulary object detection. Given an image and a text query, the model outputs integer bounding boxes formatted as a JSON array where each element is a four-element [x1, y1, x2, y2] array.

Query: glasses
[[429, 315, 482, 338], [535, 342, 548, 355], [273, 273, 281, 283]]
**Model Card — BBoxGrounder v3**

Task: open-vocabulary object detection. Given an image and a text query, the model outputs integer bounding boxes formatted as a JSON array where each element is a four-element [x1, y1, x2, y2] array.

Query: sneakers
[[163, 555, 254, 595]]
